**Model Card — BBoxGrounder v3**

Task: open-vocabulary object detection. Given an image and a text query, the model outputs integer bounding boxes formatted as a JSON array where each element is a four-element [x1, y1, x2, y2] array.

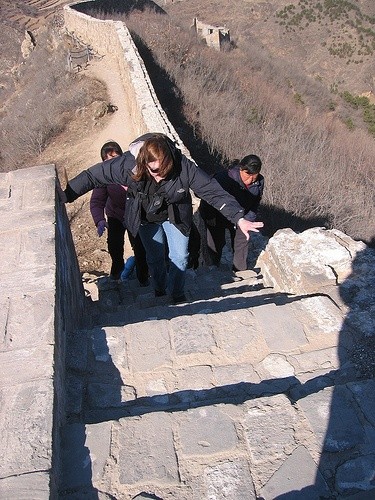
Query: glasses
[[146, 163, 160, 173]]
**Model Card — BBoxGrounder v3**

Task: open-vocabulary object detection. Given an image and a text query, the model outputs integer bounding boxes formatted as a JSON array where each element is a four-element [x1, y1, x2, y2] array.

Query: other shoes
[[155, 289, 167, 297], [121, 279, 129, 286], [173, 295, 187, 303], [141, 281, 150, 287]]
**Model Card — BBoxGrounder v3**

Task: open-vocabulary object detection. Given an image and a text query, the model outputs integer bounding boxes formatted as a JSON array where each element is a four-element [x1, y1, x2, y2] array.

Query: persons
[[55, 132, 264, 305], [90, 142, 150, 287], [197, 154, 264, 275]]
